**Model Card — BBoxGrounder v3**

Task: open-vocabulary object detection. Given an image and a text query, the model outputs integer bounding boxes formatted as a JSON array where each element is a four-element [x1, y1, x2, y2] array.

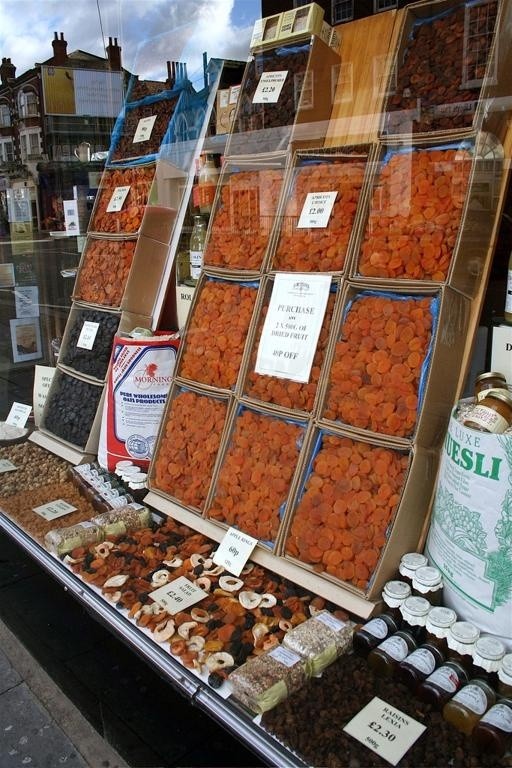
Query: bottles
[[79, 473, 110, 495], [396, 596, 430, 639], [127, 473, 149, 506], [475, 372, 508, 401], [400, 552, 429, 587], [369, 632, 417, 680], [419, 662, 469, 709], [446, 621, 479, 661], [93, 488, 126, 510], [398, 643, 443, 690], [354, 614, 397, 659], [382, 581, 410, 613], [464, 391, 512, 433], [443, 678, 495, 735], [471, 637, 506, 686], [425, 607, 456, 650], [69, 461, 99, 481], [504, 256, 512, 326], [120, 466, 141, 489], [496, 654, 512, 699], [101, 493, 134, 514], [87, 479, 119, 503], [411, 565, 444, 606], [115, 460, 133, 480], [73, 468, 106, 487], [473, 697, 512, 759]]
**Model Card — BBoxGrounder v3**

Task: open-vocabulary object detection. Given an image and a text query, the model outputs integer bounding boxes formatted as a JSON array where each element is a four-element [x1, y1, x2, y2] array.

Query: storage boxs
[[86, 157, 190, 243], [225, 34, 342, 156], [349, 135, 502, 301], [71, 234, 175, 315], [376, 0, 512, 142], [124, 16, 227, 104], [202, 158, 291, 271], [203, 398, 314, 555], [172, 269, 264, 394], [56, 303, 154, 381], [279, 421, 439, 596], [316, 279, 472, 445], [240, 275, 344, 419], [148, 384, 235, 516], [267, 148, 375, 273], [104, 90, 209, 168], [35, 366, 107, 450]]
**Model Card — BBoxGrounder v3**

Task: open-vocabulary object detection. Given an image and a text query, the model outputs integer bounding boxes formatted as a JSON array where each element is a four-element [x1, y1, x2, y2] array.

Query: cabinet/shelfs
[[0, 403, 512, 768]]
